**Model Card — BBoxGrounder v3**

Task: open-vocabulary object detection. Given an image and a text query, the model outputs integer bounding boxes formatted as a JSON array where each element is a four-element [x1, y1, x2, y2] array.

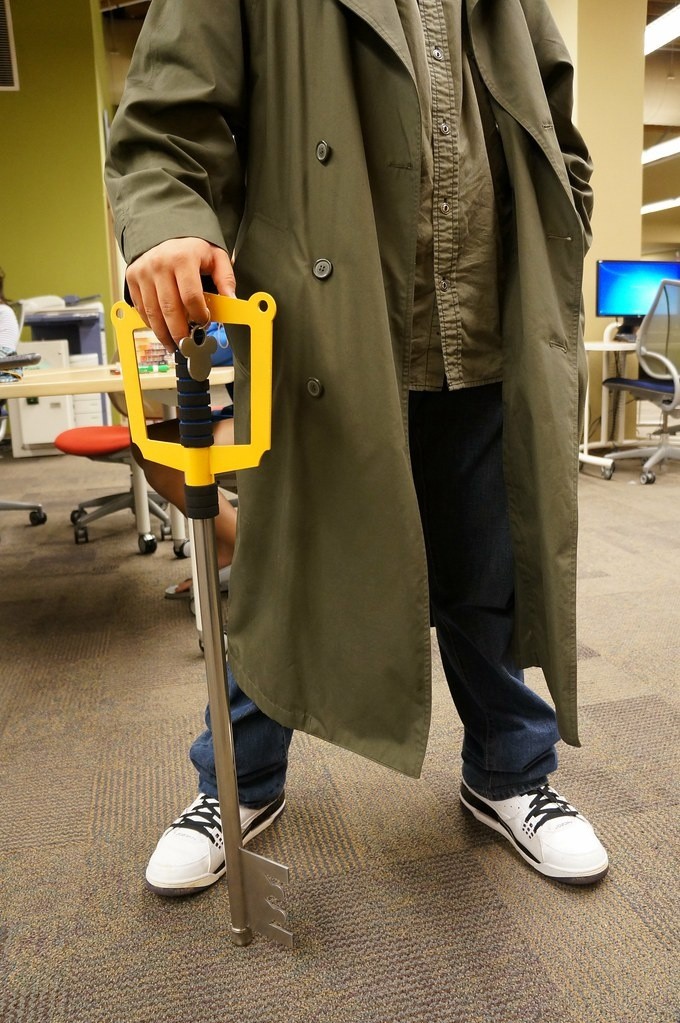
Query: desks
[[0, 365, 236, 558], [579, 340, 680, 470]]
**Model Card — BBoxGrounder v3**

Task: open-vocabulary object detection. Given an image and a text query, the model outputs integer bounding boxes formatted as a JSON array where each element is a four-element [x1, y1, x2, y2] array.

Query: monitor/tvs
[[595, 260, 679, 320]]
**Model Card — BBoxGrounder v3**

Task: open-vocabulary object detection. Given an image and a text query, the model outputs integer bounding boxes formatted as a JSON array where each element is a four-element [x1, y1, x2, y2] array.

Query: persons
[[97, 1, 610, 900]]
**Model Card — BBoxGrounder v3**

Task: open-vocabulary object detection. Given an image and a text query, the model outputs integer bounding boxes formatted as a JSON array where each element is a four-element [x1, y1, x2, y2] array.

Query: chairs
[[54, 348, 172, 554], [0, 302, 48, 527], [601, 279, 680, 485]]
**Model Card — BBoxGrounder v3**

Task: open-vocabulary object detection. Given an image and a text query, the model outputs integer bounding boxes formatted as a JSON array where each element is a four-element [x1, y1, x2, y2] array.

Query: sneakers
[[145, 789, 287, 898], [459, 777, 610, 887]]
